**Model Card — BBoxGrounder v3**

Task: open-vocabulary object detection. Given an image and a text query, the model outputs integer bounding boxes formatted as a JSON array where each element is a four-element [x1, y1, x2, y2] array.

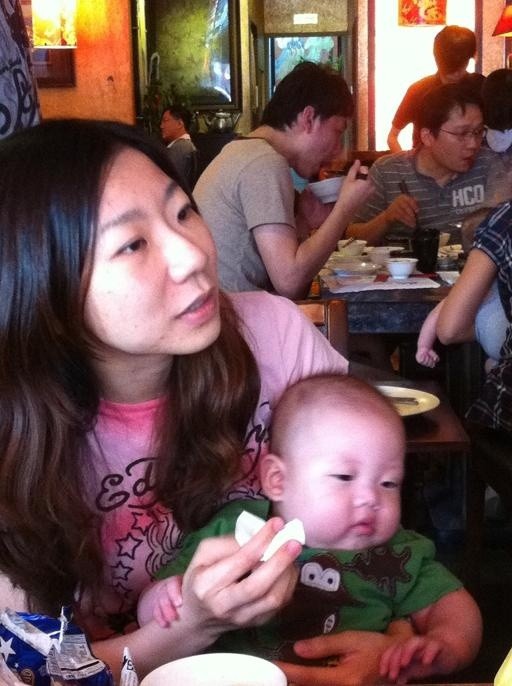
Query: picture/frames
[[143, 0, 244, 113]]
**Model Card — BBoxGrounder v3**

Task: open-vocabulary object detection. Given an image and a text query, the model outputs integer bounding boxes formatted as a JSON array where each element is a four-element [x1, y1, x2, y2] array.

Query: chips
[[230, 509, 306, 565]]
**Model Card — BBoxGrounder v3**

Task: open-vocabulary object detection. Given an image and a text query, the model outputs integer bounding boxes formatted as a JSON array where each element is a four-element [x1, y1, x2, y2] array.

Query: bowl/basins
[[384, 257, 421, 280], [309, 175, 353, 206], [334, 238, 365, 252], [139, 650, 289, 686], [368, 246, 405, 265]]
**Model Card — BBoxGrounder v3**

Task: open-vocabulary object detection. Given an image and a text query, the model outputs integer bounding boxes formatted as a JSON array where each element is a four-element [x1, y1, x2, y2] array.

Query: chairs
[[287, 298, 471, 544]]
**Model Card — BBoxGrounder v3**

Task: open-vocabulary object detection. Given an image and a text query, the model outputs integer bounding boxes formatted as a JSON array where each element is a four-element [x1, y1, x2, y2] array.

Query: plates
[[438, 243, 462, 258], [323, 252, 382, 277], [369, 385, 441, 416]]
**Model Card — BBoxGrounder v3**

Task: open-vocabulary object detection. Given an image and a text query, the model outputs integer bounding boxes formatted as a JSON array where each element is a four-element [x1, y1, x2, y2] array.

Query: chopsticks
[[340, 233, 358, 249], [399, 177, 423, 227], [325, 170, 370, 181]]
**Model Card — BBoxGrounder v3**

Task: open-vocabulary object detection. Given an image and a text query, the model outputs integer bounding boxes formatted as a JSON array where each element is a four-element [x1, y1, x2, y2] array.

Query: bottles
[[412, 226, 439, 276]]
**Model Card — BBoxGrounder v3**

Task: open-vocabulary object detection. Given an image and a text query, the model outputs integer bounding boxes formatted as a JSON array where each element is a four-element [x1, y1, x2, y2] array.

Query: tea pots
[[202, 108, 243, 133]]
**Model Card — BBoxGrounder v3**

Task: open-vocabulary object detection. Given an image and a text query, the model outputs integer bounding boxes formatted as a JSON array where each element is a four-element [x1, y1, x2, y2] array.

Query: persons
[[386, 22, 512, 153], [135, 370, 483, 684], [1, 114, 350, 683], [414, 271, 506, 376], [191, 58, 396, 374], [0, 1, 42, 134], [436, 195, 510, 458], [159, 102, 205, 197], [344, 80, 505, 360]]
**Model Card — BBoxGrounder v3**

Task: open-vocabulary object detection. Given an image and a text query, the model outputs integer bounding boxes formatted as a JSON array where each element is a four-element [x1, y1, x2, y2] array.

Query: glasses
[[438, 125, 487, 144]]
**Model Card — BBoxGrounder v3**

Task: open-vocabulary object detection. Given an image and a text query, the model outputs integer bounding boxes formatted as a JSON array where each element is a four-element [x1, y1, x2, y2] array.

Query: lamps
[[492, 2, 512, 69]]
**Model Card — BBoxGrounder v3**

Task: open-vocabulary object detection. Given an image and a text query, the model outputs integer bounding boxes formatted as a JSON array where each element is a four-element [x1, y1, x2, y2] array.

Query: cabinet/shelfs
[[193, 131, 238, 176]]
